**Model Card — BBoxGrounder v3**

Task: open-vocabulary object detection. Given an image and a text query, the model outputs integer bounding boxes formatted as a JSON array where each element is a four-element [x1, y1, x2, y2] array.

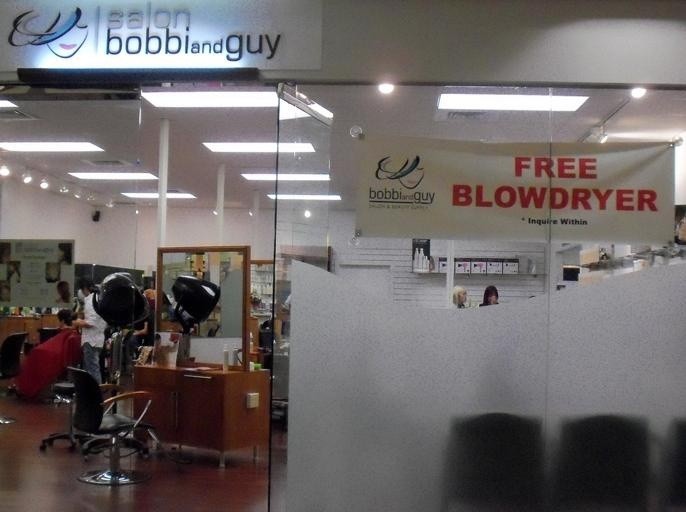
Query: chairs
[[657, 419, 686, 512], [551, 414, 650, 512], [0, 327, 158, 485], [441, 412, 550, 512]]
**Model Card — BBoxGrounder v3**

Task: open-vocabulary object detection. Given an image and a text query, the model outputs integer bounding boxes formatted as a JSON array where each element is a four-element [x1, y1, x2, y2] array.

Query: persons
[[452, 285, 468, 309], [55, 280, 72, 304], [100, 285, 154, 382], [46, 264, 58, 283], [258, 306, 290, 369], [479, 285, 499, 306], [7, 262, 21, 284], [13, 309, 74, 399], [0, 285, 10, 302], [152, 333, 180, 369], [69, 277, 108, 385]]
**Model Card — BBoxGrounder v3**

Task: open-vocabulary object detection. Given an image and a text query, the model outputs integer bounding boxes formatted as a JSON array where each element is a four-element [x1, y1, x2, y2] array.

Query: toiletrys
[[223, 343, 229, 374], [231, 342, 238, 367]]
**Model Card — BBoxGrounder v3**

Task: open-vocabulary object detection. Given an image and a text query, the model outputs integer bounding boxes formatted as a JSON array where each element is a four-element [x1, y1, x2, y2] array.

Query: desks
[[133, 362, 271, 469]]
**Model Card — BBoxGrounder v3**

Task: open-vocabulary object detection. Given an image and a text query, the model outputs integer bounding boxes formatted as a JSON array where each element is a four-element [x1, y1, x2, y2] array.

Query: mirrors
[[153, 245, 251, 372]]
